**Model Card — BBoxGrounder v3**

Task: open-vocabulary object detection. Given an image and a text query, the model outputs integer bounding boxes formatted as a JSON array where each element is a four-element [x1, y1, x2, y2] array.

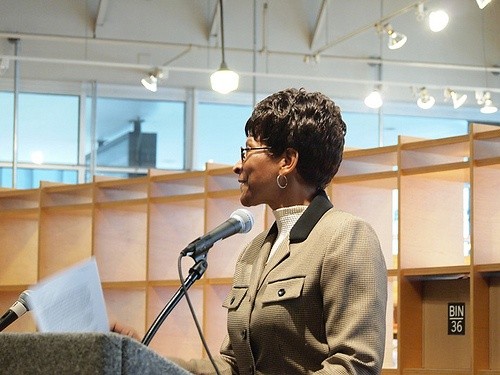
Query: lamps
[[210, 0, 239, 93], [416, 3, 448, 32], [141, 66, 163, 92], [412, 86, 435, 109], [443, 87, 468, 109], [480, 8, 498, 114], [374, 22, 407, 49]]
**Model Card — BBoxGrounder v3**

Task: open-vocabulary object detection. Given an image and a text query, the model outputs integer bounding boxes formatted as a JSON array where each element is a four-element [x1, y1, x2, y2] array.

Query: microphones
[[179, 209, 255, 256], [0, 289, 34, 332]]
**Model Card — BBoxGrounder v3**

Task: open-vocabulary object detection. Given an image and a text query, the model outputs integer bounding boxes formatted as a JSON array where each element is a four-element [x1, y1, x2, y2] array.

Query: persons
[[110, 87, 388, 375]]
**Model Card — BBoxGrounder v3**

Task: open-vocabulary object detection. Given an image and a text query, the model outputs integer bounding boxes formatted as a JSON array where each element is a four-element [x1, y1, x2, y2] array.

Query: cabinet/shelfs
[[0, 121, 500, 375]]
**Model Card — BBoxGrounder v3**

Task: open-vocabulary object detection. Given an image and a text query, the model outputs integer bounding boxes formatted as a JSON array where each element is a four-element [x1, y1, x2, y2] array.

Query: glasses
[[241, 146, 276, 162]]
